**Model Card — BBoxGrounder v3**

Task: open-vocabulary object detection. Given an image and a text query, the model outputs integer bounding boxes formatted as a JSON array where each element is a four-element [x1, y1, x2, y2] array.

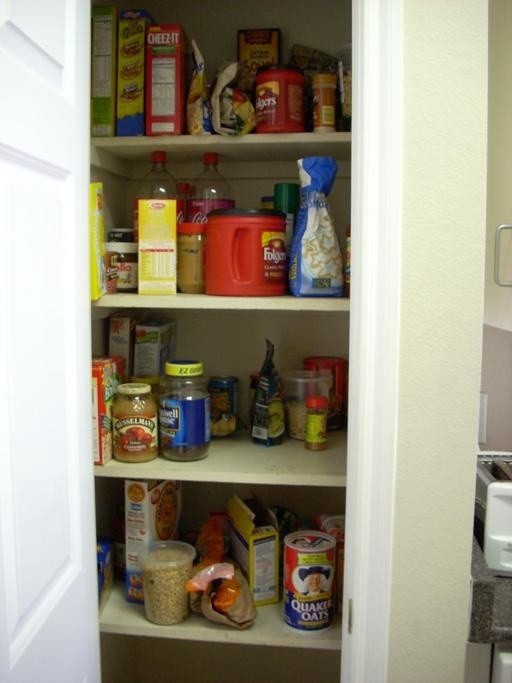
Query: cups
[[139, 540, 195, 626], [281, 372, 327, 441]]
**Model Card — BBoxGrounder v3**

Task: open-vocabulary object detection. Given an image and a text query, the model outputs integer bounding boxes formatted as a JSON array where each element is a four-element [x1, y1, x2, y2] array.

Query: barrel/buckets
[[204, 208, 286, 296]]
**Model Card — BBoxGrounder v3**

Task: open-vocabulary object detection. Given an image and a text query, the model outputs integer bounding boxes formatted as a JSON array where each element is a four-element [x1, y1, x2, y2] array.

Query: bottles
[[312, 73, 338, 129], [111, 356, 211, 463], [136, 147, 232, 293], [305, 395, 329, 450]]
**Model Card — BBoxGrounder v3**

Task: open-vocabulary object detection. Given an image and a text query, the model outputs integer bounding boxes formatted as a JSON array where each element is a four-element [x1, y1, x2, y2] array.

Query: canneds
[[177, 223, 208, 293], [209, 375, 239, 437], [112, 383, 158, 463], [158, 361, 211, 462]]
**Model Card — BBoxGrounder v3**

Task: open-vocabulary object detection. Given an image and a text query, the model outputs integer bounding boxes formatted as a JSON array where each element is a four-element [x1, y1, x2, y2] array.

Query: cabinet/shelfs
[[87, 130, 351, 650]]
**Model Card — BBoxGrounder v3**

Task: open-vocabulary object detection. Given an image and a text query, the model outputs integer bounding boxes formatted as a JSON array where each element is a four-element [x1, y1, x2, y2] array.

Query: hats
[[299, 566, 330, 580]]
